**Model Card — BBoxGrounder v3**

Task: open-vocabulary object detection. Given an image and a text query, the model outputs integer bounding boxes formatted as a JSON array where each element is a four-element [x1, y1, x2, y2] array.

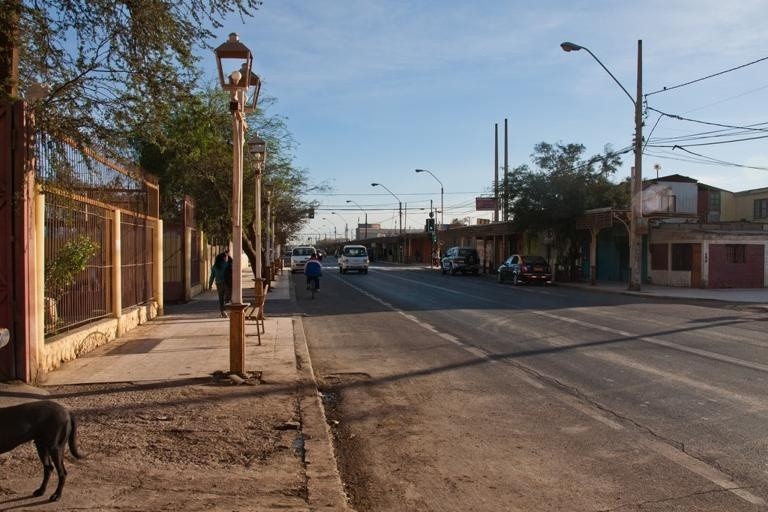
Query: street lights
[[370, 182, 402, 235], [415, 168, 444, 229], [560, 42, 646, 292], [309, 209, 349, 248], [211, 22, 279, 377], [346, 200, 368, 239]]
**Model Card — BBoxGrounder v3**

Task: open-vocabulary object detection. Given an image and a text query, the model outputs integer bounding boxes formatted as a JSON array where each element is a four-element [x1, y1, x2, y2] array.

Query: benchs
[[224, 296, 265, 346]]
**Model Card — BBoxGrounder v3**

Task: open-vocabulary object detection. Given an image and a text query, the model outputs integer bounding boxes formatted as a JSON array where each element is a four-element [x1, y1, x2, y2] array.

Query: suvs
[[439, 246, 481, 275]]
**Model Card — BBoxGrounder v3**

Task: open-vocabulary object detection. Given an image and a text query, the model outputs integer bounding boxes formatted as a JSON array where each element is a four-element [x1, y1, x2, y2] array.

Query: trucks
[[337, 244, 370, 274], [289, 246, 318, 273]]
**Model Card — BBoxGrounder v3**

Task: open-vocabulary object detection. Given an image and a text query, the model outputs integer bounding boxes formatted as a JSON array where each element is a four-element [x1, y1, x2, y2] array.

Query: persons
[[223, 250, 233, 303], [302, 254, 323, 292], [431, 249, 439, 267], [207, 252, 233, 318]]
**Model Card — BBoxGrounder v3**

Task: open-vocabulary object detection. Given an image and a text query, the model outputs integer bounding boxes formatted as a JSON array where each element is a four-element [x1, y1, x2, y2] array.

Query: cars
[[497, 253, 553, 286], [318, 249, 327, 262]]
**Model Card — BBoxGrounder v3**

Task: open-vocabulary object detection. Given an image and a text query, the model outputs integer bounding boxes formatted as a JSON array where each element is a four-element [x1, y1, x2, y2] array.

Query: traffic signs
[[432, 233, 437, 243]]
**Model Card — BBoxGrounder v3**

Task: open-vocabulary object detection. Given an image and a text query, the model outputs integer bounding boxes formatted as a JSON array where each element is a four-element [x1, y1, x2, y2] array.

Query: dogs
[[0, 401, 86, 502]]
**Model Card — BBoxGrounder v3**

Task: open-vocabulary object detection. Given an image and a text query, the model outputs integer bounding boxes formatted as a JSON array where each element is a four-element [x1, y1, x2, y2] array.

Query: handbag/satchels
[[225, 265, 232, 287]]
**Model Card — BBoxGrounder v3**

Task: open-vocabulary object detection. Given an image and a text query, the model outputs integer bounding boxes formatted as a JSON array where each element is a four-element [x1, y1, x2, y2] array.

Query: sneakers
[[316, 289, 320, 291], [221, 311, 228, 318], [225, 301, 232, 305], [307, 285, 310, 290]]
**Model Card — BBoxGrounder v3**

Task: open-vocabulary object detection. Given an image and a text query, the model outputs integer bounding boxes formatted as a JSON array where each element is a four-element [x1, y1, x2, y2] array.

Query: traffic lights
[[426, 218, 435, 232]]
